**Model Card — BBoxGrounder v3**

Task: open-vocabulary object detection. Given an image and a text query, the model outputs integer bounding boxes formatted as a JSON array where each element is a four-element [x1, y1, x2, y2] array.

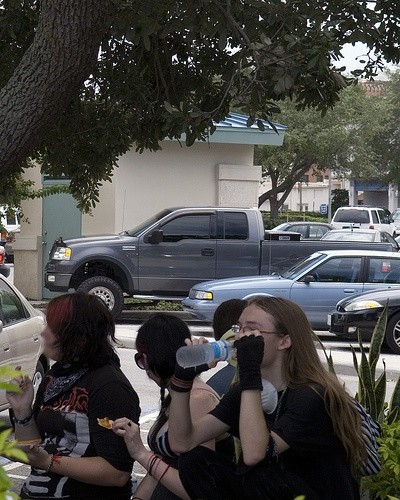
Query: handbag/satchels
[[349, 397, 383, 475]]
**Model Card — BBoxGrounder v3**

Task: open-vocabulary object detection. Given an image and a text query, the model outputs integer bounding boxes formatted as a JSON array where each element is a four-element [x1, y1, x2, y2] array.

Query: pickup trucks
[[43, 204, 393, 319]]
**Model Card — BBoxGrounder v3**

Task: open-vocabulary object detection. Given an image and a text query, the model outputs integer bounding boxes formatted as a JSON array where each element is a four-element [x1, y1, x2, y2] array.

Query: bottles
[[176, 340, 238, 369]]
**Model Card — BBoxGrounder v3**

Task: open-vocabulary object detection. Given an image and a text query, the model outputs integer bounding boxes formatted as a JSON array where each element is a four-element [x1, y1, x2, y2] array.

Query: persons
[[7, 291, 142, 500], [168, 298, 363, 500], [111, 313, 195, 500]]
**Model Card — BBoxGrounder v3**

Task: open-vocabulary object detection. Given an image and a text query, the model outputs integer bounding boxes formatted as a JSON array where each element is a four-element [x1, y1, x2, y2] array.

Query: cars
[[0, 243, 10, 278], [390, 208, 400, 234], [180, 249, 400, 341], [272, 222, 337, 239], [0, 272, 50, 428], [326, 287, 400, 356], [320, 227, 400, 252]]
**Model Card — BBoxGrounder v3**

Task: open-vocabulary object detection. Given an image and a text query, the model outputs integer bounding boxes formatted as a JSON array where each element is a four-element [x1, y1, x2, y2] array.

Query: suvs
[[330, 206, 397, 237]]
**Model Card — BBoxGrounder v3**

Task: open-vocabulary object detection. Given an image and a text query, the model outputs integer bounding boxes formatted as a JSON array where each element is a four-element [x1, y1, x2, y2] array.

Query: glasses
[[135, 352, 152, 370], [230, 324, 290, 337]]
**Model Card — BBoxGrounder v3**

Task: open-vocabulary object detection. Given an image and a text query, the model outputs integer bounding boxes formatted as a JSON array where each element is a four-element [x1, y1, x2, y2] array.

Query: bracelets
[[132, 497, 143, 500], [146, 454, 171, 482], [12, 409, 33, 424], [47, 451, 61, 471]]
